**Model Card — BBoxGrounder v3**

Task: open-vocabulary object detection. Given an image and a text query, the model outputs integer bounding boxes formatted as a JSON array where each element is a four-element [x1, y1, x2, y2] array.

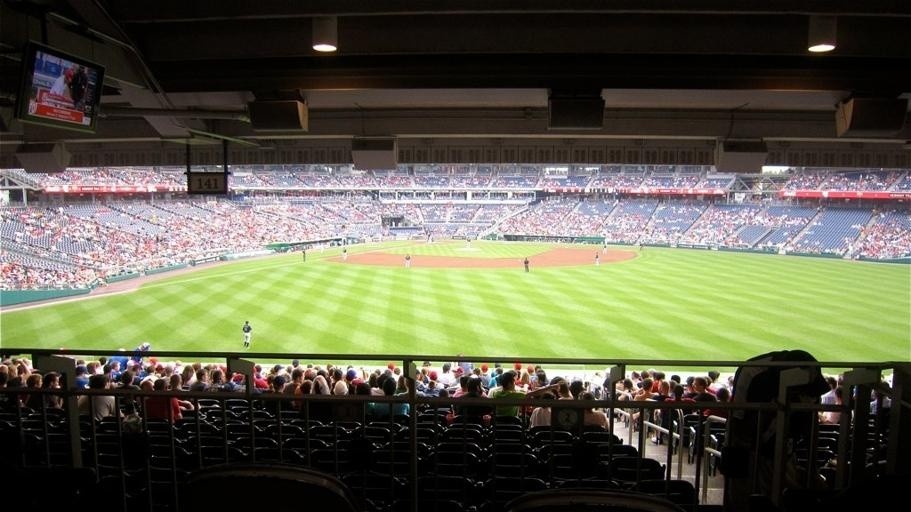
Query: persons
[[50, 61, 88, 110], [718, 349, 831, 508]]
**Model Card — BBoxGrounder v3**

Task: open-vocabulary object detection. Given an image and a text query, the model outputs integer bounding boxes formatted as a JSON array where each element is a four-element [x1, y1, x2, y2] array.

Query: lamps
[[309, 14, 338, 53], [807, 11, 838, 54]]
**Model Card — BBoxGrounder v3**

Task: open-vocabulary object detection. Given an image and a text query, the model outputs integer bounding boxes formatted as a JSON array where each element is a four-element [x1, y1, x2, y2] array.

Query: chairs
[[679, 162, 911, 262], [3, 389, 911, 512], [0, 165, 260, 285], [260, 162, 679, 244]]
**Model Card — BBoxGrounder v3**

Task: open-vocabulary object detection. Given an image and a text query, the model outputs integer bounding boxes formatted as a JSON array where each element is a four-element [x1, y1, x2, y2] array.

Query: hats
[[65, 67, 75, 82], [430, 363, 534, 379], [274, 360, 395, 385]]
